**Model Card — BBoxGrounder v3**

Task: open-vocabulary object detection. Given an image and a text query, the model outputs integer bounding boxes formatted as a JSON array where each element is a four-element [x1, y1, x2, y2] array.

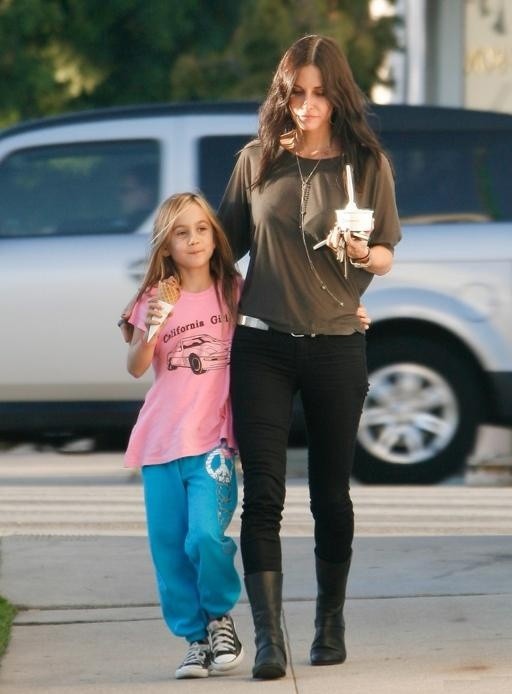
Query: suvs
[[1, 95, 512, 489]]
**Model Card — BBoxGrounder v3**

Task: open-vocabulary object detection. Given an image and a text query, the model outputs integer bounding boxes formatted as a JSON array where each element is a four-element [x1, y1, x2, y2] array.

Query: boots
[[311, 546, 352, 666], [243, 571, 286, 679]]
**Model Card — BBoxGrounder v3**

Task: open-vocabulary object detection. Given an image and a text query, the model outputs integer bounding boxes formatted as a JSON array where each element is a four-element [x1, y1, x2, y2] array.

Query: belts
[[235, 314, 316, 337]]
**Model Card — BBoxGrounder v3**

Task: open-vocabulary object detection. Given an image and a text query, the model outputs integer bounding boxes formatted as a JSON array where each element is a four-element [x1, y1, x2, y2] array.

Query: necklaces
[[294, 130, 324, 215]]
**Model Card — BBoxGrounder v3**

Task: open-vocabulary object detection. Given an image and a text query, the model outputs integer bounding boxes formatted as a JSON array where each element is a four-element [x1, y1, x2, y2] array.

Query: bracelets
[[349, 255, 372, 271], [352, 246, 373, 261]]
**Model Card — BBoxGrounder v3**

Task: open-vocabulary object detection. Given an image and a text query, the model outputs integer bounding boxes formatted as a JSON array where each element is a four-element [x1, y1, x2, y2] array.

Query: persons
[[117, 32, 402, 680], [122, 186, 374, 682], [112, 170, 159, 232]]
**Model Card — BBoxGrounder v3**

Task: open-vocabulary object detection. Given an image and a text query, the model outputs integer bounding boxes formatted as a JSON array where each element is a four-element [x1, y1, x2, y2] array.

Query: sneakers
[[175, 641, 212, 678], [207, 614, 244, 670]]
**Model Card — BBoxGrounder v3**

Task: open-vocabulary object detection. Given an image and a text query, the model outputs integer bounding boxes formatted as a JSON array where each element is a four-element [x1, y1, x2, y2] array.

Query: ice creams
[[145, 276, 182, 343]]
